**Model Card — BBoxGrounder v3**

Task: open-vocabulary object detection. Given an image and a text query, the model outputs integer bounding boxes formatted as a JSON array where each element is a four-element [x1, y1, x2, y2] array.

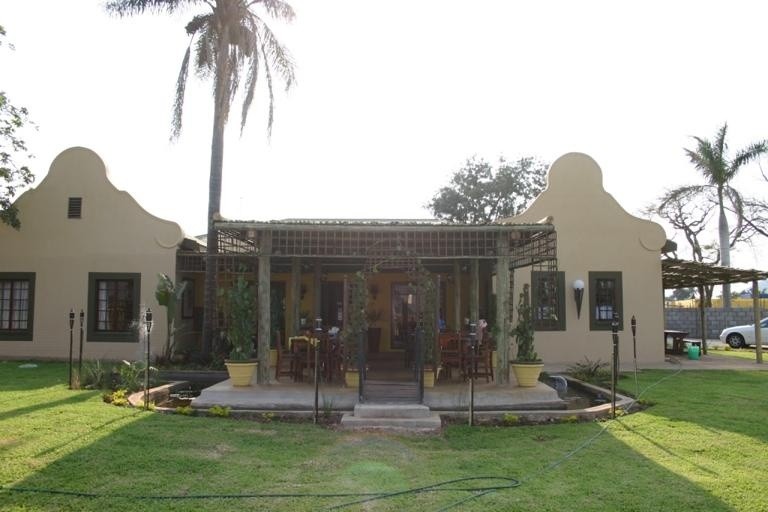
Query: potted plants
[[213, 263, 260, 386], [342, 272, 384, 386], [509, 284, 545, 387], [405, 267, 443, 387]]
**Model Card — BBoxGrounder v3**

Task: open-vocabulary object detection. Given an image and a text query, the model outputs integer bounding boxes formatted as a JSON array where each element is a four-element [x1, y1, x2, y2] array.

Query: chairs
[[462, 327, 494, 384], [272, 322, 346, 382]]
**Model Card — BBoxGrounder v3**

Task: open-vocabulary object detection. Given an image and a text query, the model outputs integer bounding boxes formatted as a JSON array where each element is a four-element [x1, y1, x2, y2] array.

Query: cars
[[719, 314, 768, 349]]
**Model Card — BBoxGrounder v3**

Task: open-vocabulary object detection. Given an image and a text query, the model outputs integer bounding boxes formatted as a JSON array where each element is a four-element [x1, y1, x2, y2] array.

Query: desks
[[664, 330, 688, 354]]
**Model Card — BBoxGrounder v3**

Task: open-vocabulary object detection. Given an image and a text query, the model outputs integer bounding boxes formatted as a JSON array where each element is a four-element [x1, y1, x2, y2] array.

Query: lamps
[[572, 280, 584, 320]]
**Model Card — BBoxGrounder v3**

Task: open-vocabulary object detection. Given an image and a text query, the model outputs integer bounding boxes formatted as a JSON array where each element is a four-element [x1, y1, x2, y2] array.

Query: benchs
[[682, 339, 701, 357]]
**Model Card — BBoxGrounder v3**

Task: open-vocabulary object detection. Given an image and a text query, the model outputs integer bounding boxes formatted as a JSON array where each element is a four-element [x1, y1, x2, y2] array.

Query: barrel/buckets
[[688, 346, 700, 359]]
